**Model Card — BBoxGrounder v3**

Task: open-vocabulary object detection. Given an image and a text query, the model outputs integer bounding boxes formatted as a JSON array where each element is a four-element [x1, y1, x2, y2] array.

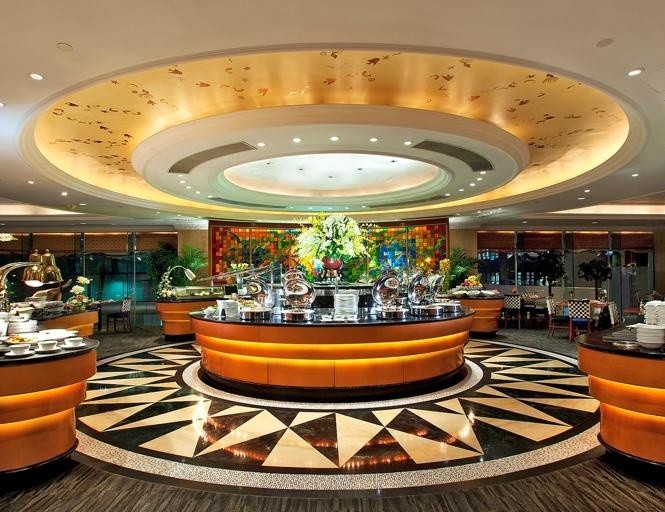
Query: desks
[[89, 299, 119, 331]]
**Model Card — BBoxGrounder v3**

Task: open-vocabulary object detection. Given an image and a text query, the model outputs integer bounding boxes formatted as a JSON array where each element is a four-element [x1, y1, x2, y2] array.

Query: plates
[[637, 324, 665, 349], [4, 350, 34, 357], [35, 346, 60, 353], [61, 343, 86, 350], [216, 299, 239, 321], [0, 316, 79, 345]]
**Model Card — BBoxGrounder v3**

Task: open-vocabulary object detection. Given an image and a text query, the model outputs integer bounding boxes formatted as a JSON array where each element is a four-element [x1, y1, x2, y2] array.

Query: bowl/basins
[[38, 341, 56, 349], [9, 301, 47, 309], [453, 290, 496, 298], [9, 344, 30, 353], [64, 337, 82, 346], [202, 307, 217, 315]]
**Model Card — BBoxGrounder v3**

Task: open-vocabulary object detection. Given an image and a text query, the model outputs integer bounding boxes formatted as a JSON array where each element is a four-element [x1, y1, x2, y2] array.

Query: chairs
[[106, 297, 132, 332], [498, 287, 620, 348]]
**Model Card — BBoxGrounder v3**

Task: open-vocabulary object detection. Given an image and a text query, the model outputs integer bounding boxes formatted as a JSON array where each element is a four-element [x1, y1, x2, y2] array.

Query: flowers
[[295, 211, 375, 284], [63, 274, 95, 312], [157, 272, 178, 298]]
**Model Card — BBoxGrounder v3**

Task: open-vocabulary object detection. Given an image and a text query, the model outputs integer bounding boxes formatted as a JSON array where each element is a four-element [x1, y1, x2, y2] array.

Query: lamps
[[0, 248, 63, 297], [164, 264, 195, 287]]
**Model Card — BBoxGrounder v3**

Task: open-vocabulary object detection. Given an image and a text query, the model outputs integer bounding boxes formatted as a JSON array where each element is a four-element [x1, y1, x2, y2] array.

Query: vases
[[321, 255, 342, 268]]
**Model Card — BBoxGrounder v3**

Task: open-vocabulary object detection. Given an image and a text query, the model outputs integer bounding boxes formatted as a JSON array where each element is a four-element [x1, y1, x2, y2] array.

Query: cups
[[320, 314, 358, 323]]
